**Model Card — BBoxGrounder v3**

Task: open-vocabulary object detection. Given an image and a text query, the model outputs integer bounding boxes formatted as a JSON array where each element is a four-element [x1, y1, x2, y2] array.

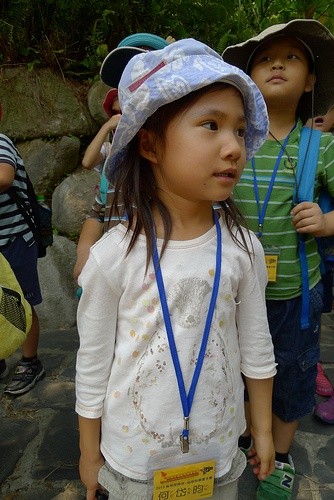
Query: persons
[[74, 86, 121, 289], [102, 33, 174, 234], [74, 38, 279, 500], [224, 18, 334, 500], [0, 134, 46, 395]]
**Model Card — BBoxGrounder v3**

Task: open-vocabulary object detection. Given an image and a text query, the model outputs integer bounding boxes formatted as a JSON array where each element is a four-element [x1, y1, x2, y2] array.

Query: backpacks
[[7, 172, 54, 258]]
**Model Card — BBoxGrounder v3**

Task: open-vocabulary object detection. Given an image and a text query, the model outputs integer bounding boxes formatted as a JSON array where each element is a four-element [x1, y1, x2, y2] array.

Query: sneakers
[[314, 397, 334, 423], [4, 360, 46, 394], [315, 362, 334, 396], [0, 360, 9, 378], [256, 454, 295, 500]]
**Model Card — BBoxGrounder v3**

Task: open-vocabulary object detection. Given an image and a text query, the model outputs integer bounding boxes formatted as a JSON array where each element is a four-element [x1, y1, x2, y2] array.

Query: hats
[[118, 33, 169, 50], [105, 38, 270, 193], [221, 19, 334, 116], [103, 88, 121, 120]]
